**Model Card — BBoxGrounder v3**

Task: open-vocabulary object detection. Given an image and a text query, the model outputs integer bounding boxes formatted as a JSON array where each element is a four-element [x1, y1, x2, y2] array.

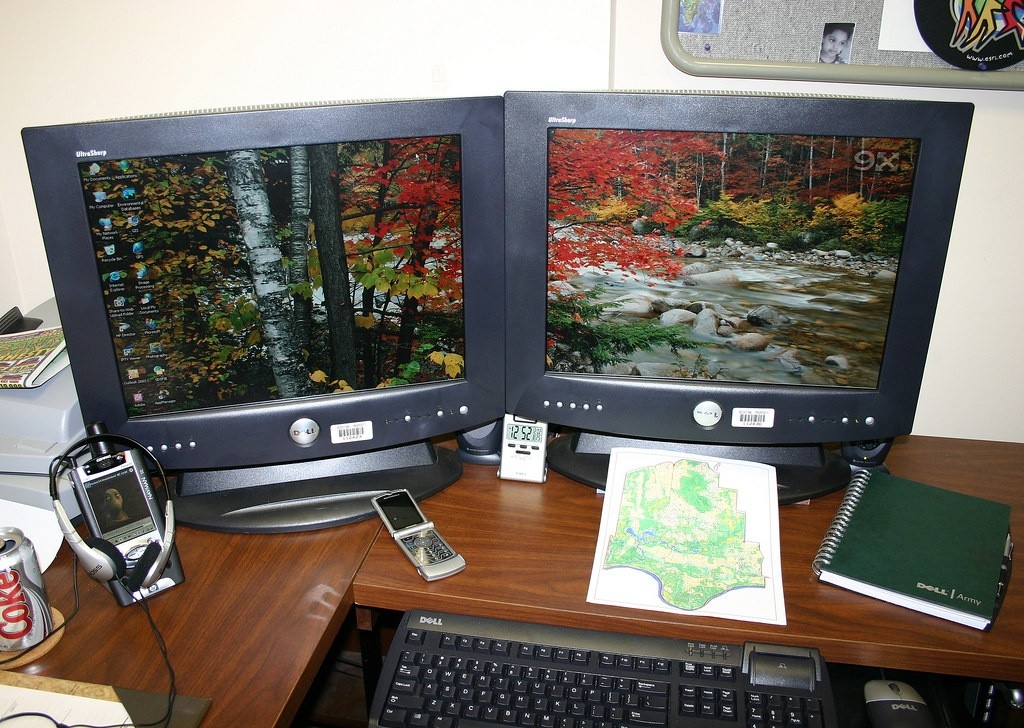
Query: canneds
[[0, 527, 54, 652]]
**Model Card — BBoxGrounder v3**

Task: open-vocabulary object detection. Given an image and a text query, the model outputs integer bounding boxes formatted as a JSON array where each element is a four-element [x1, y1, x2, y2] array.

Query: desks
[[0, 436, 433, 728], [352, 434, 1023, 728]]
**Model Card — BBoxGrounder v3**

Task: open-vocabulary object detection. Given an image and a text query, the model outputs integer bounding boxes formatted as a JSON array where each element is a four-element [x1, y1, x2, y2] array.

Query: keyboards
[[367, 608, 839, 728]]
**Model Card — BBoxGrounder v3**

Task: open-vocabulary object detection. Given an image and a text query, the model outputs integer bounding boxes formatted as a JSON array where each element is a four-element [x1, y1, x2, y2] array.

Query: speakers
[[454, 418, 504, 465], [840, 437, 895, 476]]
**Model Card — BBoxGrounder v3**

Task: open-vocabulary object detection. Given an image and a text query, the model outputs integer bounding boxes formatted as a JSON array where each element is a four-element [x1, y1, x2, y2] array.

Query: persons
[[820, 23, 856, 66]]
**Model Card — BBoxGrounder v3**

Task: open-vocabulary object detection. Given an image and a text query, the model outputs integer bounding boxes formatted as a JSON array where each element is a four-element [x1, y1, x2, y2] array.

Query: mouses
[[864, 679, 936, 728]]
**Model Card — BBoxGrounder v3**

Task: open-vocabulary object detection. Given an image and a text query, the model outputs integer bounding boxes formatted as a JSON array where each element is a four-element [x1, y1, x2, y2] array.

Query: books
[[812, 469, 1016, 633]]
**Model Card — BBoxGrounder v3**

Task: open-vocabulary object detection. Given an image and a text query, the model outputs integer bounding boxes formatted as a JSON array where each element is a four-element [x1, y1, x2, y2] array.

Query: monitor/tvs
[[21, 95, 508, 535], [505, 88, 975, 507]]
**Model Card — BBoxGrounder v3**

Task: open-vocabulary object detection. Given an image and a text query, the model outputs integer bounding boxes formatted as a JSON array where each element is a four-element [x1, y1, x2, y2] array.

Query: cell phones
[[372, 489, 467, 582]]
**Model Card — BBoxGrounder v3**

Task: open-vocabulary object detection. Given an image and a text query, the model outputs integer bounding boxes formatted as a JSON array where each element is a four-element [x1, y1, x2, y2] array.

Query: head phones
[[47, 430, 174, 592]]
